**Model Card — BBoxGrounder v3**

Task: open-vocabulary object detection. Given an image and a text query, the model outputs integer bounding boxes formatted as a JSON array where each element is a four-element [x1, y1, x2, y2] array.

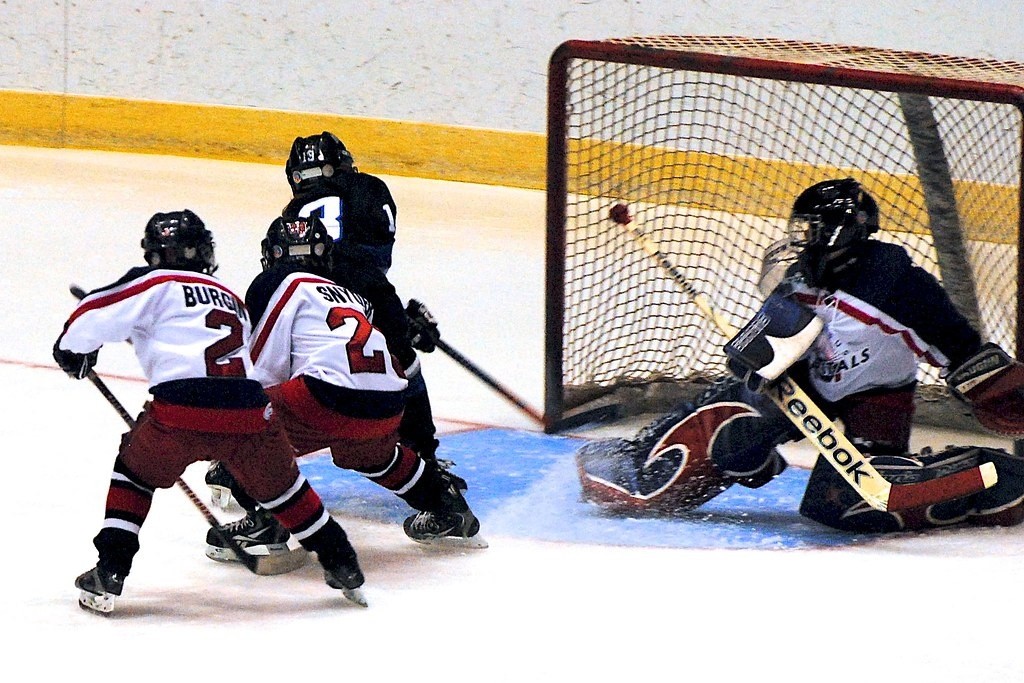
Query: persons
[[205, 132, 470, 511], [205, 217, 488, 562], [53, 209, 367, 615], [576, 178, 1024, 535]]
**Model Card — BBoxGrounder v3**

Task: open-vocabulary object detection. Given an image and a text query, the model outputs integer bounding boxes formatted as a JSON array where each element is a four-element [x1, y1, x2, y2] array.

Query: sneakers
[[206, 507, 290, 561], [403, 484, 488, 549], [74, 558, 130, 615], [317, 538, 369, 607], [204, 460, 234, 510]]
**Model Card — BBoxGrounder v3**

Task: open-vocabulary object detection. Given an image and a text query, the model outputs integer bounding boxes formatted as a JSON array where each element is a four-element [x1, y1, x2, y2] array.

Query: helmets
[[141, 209, 219, 276], [788, 178, 879, 263], [261, 216, 333, 278], [285, 131, 358, 198]]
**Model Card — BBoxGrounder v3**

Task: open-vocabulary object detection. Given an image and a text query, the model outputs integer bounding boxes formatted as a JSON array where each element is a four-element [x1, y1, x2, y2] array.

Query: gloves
[[404, 299, 440, 353], [53, 343, 98, 380]]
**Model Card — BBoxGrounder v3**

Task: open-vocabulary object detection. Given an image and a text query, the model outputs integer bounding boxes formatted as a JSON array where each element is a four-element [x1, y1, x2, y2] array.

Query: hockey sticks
[[437, 339, 546, 425], [68, 284, 470, 498], [87, 367, 319, 577], [608, 203, 1000, 515]]
